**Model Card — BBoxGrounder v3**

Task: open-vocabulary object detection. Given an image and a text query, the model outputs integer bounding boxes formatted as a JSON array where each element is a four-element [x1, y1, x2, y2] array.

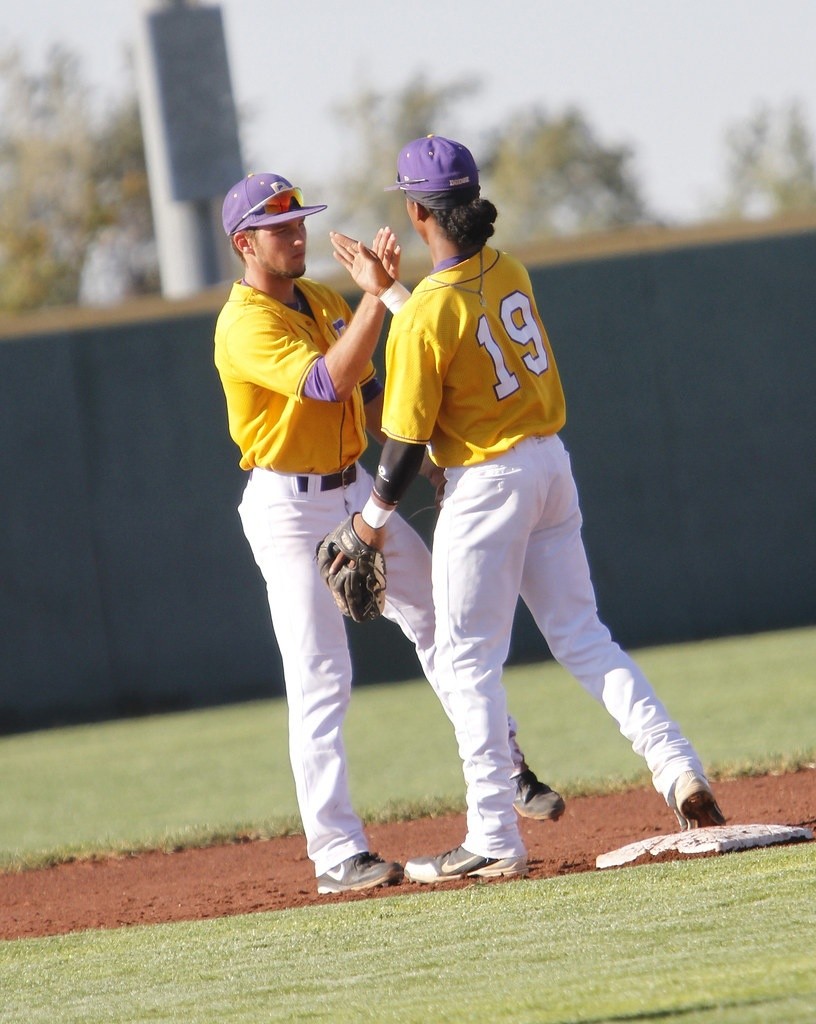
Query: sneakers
[[674, 769, 728, 830], [507, 767, 566, 823], [317, 851, 404, 895], [402, 844, 528, 886]]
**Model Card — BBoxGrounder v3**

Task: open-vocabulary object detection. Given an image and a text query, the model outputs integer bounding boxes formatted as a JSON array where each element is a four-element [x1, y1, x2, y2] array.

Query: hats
[[383, 134, 479, 192], [221, 171, 328, 237]]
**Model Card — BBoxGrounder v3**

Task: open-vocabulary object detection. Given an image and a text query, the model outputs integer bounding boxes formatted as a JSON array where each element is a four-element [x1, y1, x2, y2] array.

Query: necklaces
[[424, 245, 487, 308]]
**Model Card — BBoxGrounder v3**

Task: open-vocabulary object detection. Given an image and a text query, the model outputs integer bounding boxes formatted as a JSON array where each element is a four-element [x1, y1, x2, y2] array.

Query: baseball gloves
[[315, 511, 388, 625]]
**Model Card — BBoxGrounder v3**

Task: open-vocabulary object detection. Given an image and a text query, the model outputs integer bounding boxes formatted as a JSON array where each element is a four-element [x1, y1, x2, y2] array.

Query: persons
[[315, 134, 726, 884], [214, 173, 566, 894]]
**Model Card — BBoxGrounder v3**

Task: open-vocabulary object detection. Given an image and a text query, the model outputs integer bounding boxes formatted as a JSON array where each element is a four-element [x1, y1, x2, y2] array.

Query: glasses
[[395, 170, 429, 187], [226, 187, 305, 237]]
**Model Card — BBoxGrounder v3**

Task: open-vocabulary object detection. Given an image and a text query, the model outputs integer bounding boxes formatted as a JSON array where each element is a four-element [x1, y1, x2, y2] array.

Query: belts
[[296, 461, 356, 493]]
